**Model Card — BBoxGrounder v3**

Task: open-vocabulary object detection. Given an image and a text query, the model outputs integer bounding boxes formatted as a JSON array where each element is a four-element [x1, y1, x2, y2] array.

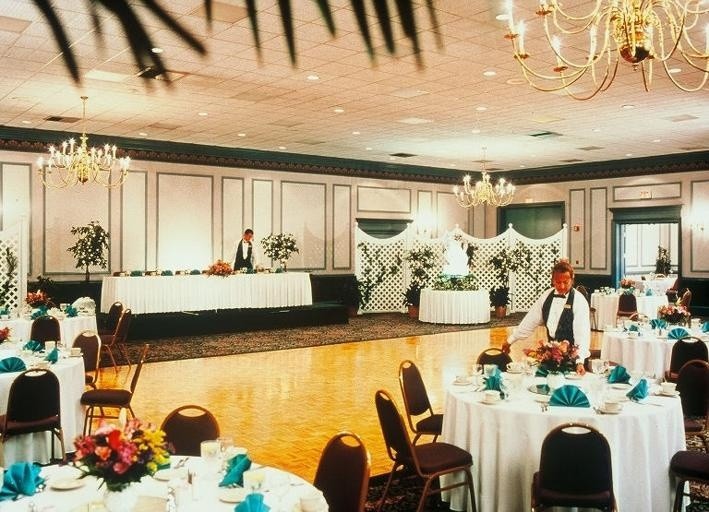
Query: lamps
[[451, 142, 517, 212], [493, 1, 709, 101], [32, 92, 133, 191]]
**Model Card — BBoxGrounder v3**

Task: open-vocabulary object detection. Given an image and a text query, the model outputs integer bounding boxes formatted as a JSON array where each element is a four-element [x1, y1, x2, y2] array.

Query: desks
[[419, 288, 490, 324], [600, 320, 709, 382], [102, 270, 312, 312], [0, 455, 329, 511]]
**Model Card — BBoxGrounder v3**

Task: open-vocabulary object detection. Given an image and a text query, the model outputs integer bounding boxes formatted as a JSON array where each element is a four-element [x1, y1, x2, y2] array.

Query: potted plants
[[402, 280, 425, 318], [489, 285, 511, 318]]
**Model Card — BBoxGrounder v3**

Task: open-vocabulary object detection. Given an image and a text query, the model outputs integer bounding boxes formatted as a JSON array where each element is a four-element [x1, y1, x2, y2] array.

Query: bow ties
[[553, 293, 566, 298]]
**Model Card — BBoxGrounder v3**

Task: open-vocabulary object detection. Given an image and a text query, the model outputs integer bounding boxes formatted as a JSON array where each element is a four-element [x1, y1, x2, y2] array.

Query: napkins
[[0, 461, 46, 501], [219, 453, 252, 489], [702, 321, 709, 333], [668, 328, 689, 340], [234, 493, 271, 511]]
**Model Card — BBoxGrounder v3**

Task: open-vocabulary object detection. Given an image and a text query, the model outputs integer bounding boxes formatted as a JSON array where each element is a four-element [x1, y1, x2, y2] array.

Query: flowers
[[434, 275, 478, 291], [523, 339, 579, 367], [74, 418, 170, 493], [260, 233, 304, 262]]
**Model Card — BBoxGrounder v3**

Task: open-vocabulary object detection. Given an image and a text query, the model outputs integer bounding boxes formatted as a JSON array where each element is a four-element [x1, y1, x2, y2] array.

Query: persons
[[231, 228, 255, 268], [500, 256, 592, 378]]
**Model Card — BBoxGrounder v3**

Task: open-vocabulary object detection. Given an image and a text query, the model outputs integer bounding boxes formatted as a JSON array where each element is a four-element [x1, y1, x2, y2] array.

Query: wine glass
[[0, 301, 84, 370]]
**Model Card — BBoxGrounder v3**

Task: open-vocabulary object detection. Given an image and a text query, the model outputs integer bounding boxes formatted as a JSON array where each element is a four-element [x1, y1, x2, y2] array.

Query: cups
[[118, 262, 287, 276], [450, 359, 680, 414], [595, 286, 708, 343], [41, 437, 325, 512]]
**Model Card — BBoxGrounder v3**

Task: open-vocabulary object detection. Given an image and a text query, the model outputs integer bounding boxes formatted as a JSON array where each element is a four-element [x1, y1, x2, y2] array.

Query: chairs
[[576, 273, 691, 332], [375, 348, 709, 510], [160, 404, 220, 456], [0, 293, 149, 460], [314, 431, 372, 510], [664, 336, 708, 382]]
